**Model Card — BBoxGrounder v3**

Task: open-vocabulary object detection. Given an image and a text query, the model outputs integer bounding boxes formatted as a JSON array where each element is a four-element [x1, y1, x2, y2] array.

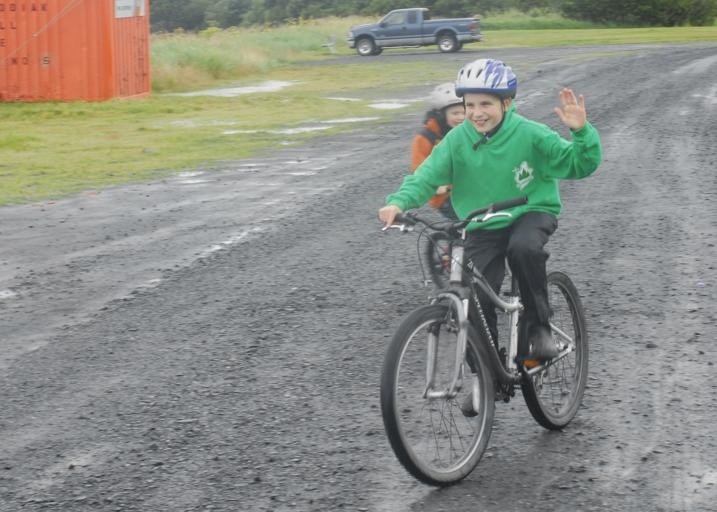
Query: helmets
[[455, 57, 518, 100], [425, 82, 462, 112]]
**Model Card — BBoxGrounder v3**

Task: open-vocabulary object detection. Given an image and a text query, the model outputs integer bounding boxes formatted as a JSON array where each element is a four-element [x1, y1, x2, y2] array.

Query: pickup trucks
[[346, 8, 484, 57]]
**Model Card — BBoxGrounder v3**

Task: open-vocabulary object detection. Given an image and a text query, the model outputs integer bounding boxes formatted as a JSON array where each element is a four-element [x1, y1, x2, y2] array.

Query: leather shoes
[[527, 322, 559, 361], [462, 399, 477, 417]]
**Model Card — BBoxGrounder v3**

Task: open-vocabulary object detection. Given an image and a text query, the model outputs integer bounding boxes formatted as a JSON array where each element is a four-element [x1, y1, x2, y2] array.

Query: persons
[[378, 60, 602, 417], [411, 80, 468, 273]]
